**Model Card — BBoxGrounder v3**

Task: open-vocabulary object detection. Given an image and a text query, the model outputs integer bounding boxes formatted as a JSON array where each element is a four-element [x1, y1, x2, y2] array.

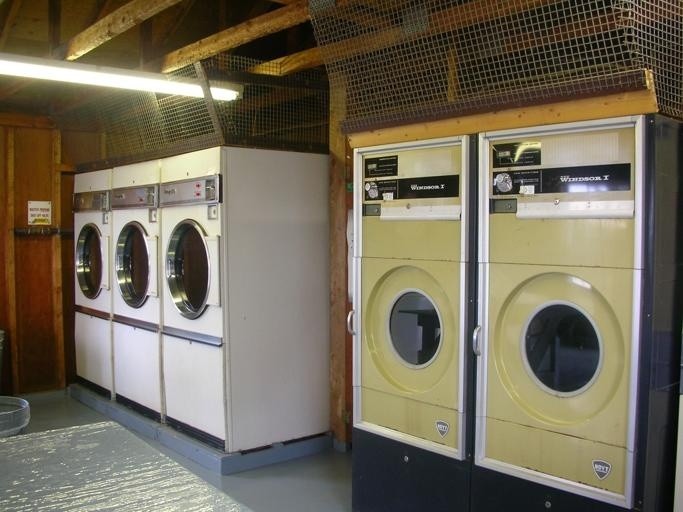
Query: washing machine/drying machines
[[67, 147, 337, 477], [474, 113, 680, 510], [352, 134, 475, 464]]
